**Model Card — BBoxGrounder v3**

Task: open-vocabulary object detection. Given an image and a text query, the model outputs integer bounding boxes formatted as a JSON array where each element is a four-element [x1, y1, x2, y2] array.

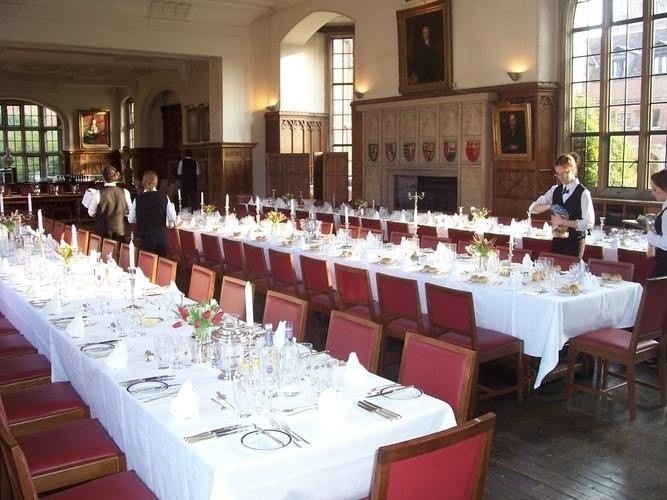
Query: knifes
[[182, 424, 249, 444], [366, 383, 413, 400], [252, 423, 284, 447], [357, 399, 401, 421]]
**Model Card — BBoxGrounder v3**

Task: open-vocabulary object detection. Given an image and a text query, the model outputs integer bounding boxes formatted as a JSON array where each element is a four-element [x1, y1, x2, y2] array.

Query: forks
[[272, 420, 310, 450]]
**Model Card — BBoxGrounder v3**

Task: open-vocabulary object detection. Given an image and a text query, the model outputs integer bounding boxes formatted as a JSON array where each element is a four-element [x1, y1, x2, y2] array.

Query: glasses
[[555, 169, 574, 177]]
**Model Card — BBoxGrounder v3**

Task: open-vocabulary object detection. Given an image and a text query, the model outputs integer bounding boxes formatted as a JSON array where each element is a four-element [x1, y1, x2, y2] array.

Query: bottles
[[55, 171, 91, 182]]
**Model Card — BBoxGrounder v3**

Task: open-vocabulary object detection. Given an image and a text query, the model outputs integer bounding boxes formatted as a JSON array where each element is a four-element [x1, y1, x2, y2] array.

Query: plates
[[381, 385, 424, 401], [240, 429, 291, 450], [0, 218, 354, 419]]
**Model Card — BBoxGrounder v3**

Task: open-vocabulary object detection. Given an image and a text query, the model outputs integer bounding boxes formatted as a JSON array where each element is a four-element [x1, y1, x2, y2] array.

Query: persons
[[527, 152, 595, 259], [638, 169, 666, 367], [408, 26, 443, 83], [87, 165, 132, 242], [126, 170, 176, 258], [84, 117, 101, 144], [499, 112, 524, 153], [176, 149, 202, 212]]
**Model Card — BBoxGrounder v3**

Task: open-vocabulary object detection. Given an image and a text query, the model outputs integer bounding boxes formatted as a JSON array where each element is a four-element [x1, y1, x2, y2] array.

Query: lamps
[[265, 105, 274, 112], [354, 92, 363, 98], [506, 71, 521, 80]]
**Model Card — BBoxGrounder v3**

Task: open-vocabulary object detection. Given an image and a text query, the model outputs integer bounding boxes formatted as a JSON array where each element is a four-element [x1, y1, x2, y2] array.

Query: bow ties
[[560, 186, 570, 194]]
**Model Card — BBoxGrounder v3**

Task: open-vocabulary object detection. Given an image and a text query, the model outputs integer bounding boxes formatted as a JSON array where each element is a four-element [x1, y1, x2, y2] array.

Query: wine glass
[[173, 208, 588, 296], [251, 189, 656, 254]]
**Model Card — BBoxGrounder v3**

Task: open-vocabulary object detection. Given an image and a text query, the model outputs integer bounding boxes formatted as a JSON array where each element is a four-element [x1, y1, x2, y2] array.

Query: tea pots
[[0, 183, 80, 197]]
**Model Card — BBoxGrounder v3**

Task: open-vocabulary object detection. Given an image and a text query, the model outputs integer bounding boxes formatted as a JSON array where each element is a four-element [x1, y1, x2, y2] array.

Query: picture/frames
[[490, 102, 532, 162], [77, 106, 112, 151], [394, 1, 452, 96]]
